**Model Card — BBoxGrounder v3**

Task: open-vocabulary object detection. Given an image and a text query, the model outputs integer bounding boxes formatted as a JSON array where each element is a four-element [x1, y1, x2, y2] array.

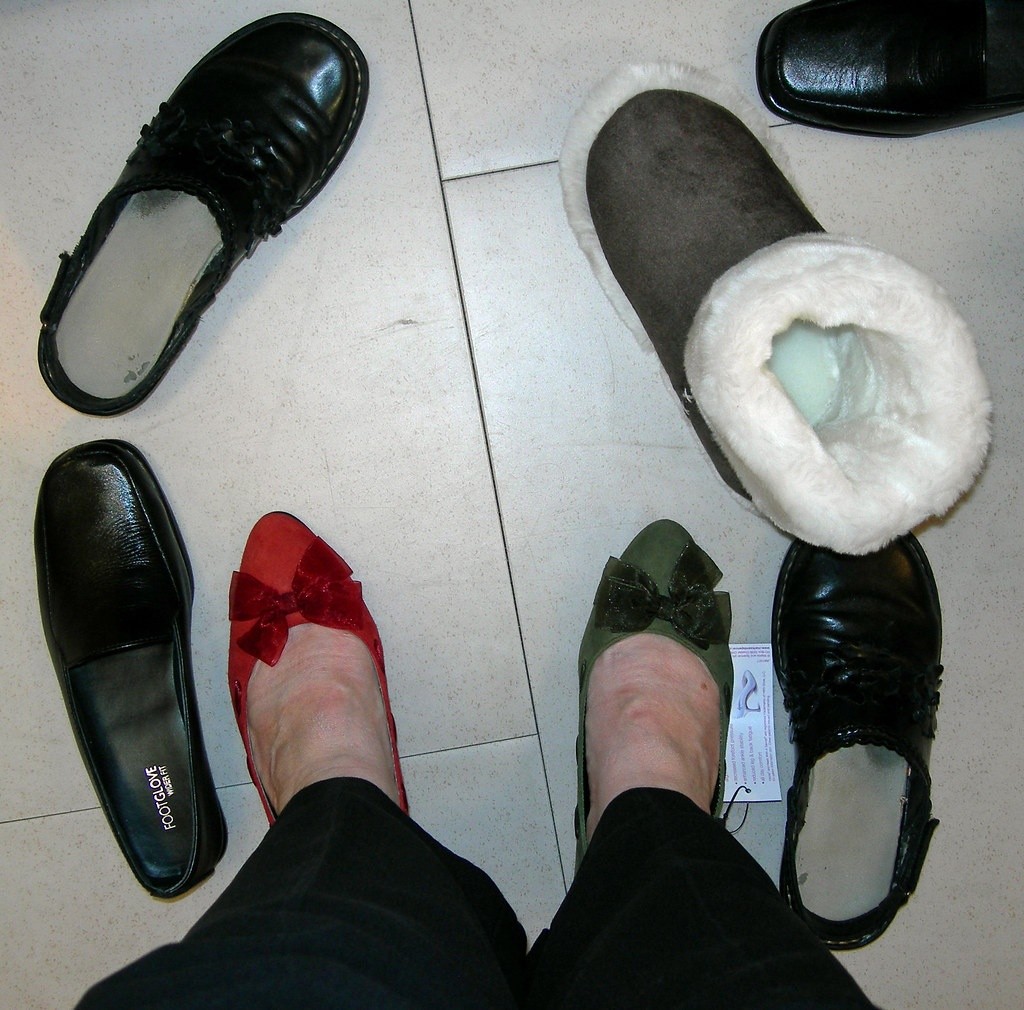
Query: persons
[[74, 511, 890, 1010]]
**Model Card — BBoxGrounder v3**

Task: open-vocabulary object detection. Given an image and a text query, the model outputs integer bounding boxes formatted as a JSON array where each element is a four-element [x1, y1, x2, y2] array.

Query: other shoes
[[572, 519, 734, 877], [561, 64, 996, 556], [226, 508, 409, 836]]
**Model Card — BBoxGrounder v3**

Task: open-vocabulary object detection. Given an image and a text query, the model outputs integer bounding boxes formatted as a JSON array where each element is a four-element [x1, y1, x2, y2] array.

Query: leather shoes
[[767, 513, 945, 944], [35, 440, 225, 899], [38, 9, 369, 418], [756, 0, 1024, 136]]
